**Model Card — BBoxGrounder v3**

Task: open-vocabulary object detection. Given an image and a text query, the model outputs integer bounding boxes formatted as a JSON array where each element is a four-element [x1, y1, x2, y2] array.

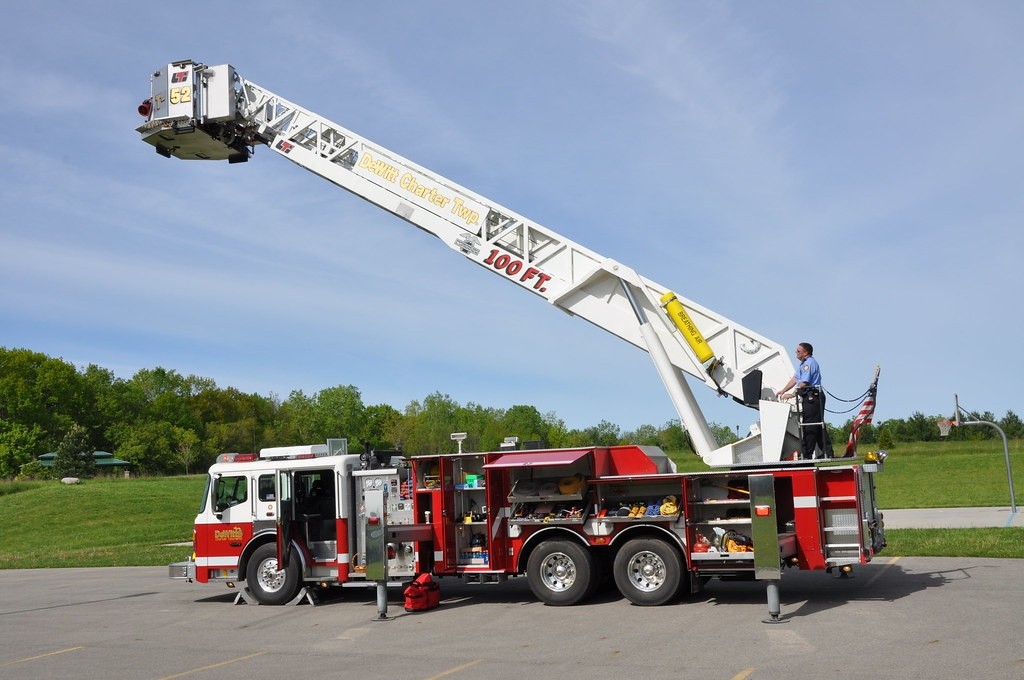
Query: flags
[[843, 369, 880, 457]]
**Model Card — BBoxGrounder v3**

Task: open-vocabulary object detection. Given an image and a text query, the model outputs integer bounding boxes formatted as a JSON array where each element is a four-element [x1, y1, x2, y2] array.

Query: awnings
[[482, 451, 590, 469]]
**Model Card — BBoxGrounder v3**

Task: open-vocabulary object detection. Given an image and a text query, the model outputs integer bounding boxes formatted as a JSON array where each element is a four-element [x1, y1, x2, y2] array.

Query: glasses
[[797, 348, 800, 353]]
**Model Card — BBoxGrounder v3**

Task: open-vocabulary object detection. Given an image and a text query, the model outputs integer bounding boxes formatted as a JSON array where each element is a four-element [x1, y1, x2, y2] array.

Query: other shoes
[[817, 453, 834, 458], [798, 455, 812, 460]]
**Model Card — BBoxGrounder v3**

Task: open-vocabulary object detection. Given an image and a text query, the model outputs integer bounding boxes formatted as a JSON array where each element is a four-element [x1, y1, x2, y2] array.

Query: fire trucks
[[135, 60, 889, 623]]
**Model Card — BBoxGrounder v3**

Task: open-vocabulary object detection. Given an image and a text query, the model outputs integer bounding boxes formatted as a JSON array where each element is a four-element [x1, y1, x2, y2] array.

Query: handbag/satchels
[[402, 573, 439, 612]]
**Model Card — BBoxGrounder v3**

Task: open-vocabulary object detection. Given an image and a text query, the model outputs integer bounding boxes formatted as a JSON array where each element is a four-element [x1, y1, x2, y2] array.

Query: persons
[[138, 98, 151, 117], [775, 342, 835, 460], [302, 480, 333, 541]]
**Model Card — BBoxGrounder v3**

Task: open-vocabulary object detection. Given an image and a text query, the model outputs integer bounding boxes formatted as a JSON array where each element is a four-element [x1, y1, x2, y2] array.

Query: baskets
[[351, 553, 366, 572]]
